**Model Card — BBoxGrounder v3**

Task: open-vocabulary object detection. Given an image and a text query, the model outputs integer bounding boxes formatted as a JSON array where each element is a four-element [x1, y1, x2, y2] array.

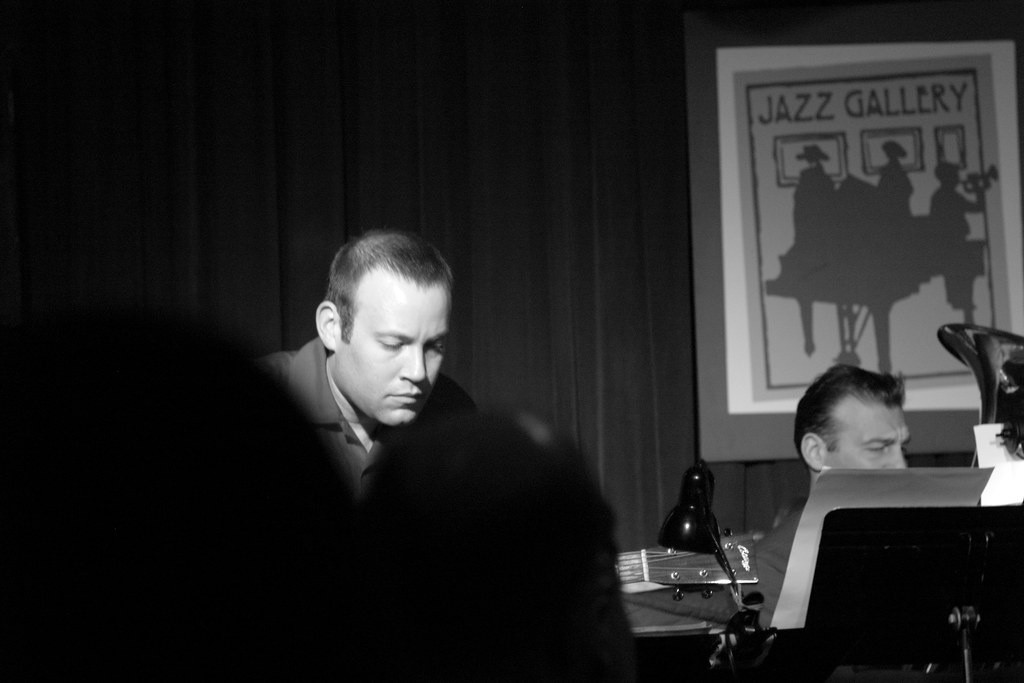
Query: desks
[[620, 530, 762, 636]]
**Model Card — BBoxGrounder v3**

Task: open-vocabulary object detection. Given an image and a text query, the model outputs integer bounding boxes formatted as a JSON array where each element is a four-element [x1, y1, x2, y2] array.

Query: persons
[[738, 363, 912, 628], [270, 228, 637, 683]]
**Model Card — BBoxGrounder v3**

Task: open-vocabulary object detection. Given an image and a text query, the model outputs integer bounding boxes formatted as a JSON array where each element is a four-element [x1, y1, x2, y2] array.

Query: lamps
[[659, 465, 743, 611]]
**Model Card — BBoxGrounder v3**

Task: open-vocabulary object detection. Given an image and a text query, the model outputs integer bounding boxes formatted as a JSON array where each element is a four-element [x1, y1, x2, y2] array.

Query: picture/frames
[[683, 0, 1024, 461]]
